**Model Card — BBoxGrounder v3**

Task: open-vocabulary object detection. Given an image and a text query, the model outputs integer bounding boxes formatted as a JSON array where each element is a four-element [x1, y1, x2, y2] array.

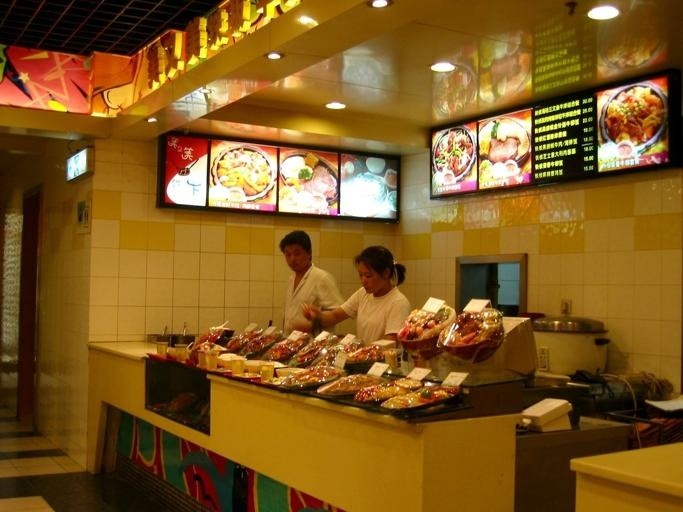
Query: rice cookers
[[531, 316, 612, 377]]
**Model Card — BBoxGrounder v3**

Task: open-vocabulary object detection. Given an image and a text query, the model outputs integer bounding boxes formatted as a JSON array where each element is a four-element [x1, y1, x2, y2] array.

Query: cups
[[196, 347, 277, 389], [174, 343, 188, 362], [156, 341, 169, 358]]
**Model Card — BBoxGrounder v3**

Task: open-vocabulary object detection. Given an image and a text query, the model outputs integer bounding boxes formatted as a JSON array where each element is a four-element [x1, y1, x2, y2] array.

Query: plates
[[598, 19, 667, 72], [211, 144, 278, 202], [166, 153, 207, 207], [600, 82, 670, 152], [205, 320, 386, 364], [433, 124, 476, 183], [280, 152, 339, 207], [478, 43, 533, 104], [477, 117, 532, 168], [282, 365, 466, 413], [430, 60, 478, 120]]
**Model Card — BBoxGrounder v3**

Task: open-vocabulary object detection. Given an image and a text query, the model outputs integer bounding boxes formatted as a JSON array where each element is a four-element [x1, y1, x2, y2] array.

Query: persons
[[301, 244, 411, 347], [279, 230, 345, 339]]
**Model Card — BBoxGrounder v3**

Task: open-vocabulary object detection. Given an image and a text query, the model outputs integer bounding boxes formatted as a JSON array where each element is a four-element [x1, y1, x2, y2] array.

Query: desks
[[567, 441, 682, 511]]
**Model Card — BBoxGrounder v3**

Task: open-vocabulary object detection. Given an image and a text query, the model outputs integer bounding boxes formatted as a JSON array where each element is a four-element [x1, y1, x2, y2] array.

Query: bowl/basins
[[438, 308, 507, 366], [396, 305, 459, 361], [365, 156, 386, 175], [383, 167, 397, 190]]
